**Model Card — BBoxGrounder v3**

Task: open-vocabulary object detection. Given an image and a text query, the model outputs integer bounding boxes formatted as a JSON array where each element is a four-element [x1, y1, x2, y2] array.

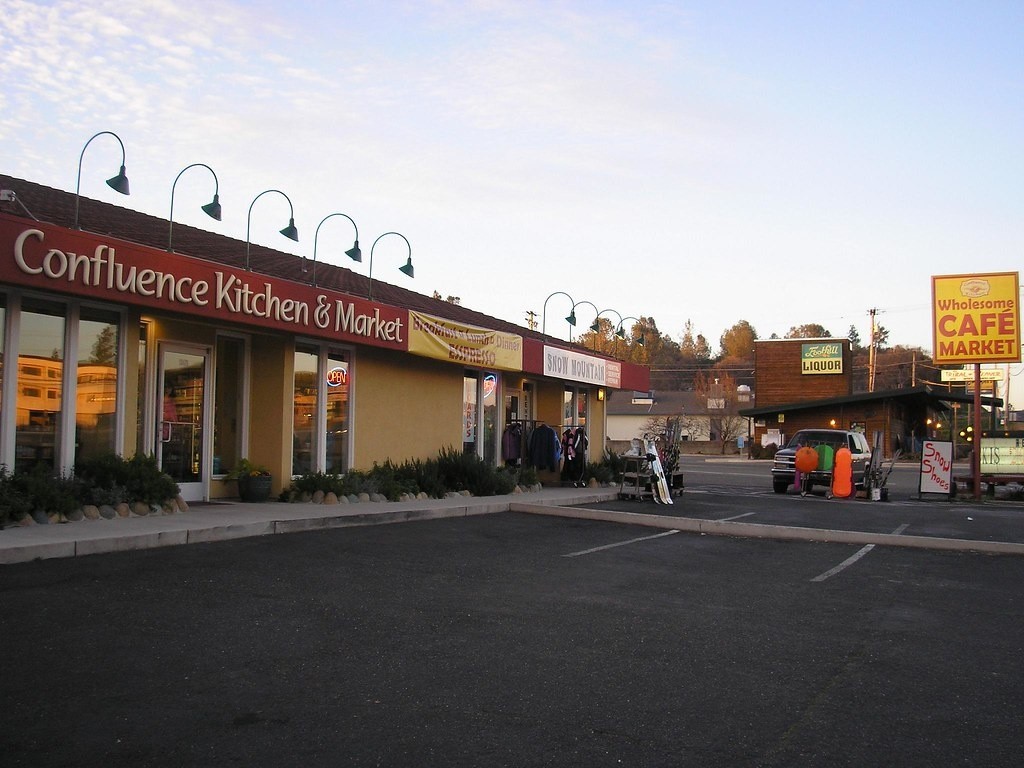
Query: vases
[[238, 476, 272, 503]]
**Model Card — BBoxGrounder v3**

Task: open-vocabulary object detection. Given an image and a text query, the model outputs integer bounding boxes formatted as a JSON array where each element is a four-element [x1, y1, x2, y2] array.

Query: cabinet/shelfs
[[619, 455, 658, 503]]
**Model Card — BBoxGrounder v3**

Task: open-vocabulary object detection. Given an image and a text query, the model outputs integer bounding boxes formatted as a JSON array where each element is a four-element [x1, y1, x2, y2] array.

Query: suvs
[[769, 427, 873, 494]]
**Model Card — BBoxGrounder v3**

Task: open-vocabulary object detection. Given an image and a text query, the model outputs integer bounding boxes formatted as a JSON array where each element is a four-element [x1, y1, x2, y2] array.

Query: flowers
[[220, 458, 271, 484]]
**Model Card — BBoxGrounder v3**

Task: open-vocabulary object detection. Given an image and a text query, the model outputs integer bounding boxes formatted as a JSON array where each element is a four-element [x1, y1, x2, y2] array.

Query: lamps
[[71, 131, 130, 232], [570, 302, 599, 350], [368, 232, 414, 300], [616, 317, 644, 360], [313, 214, 362, 285], [246, 190, 298, 271], [167, 164, 221, 253], [594, 310, 624, 355], [598, 388, 604, 400], [543, 292, 576, 343]]
